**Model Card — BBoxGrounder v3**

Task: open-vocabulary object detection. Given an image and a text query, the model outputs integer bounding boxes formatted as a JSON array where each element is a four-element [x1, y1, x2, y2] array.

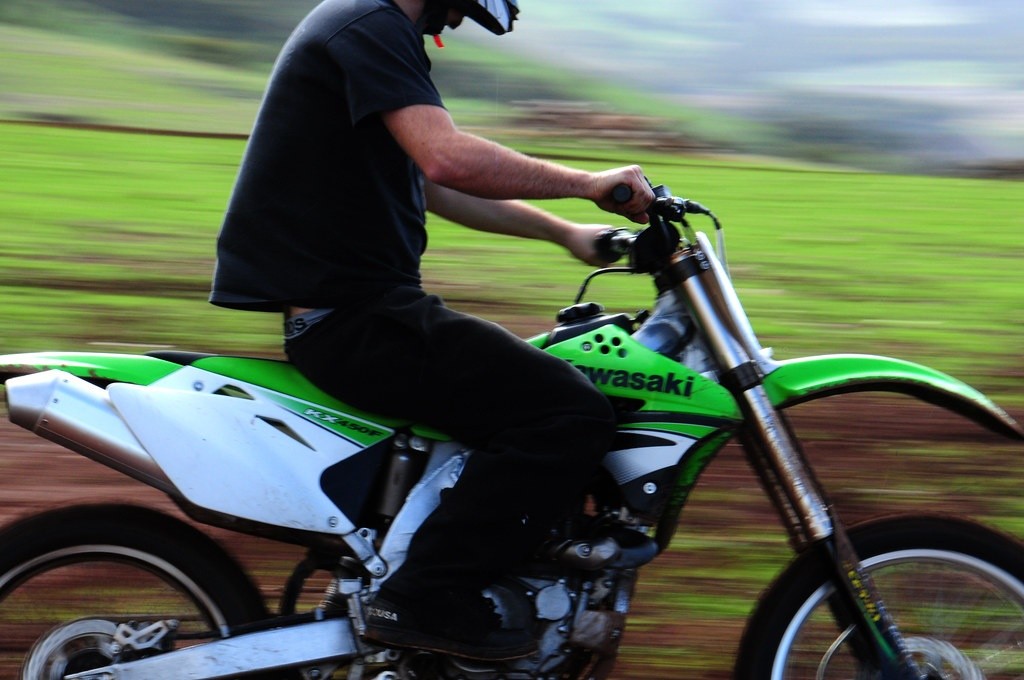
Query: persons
[[210, 1, 656, 657]]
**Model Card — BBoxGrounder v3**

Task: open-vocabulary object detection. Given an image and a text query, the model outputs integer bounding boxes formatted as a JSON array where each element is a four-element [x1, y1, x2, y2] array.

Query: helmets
[[459, 0, 520, 36]]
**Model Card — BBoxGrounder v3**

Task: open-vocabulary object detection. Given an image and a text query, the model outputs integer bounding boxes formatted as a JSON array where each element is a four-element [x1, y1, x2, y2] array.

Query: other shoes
[[362, 588, 540, 661]]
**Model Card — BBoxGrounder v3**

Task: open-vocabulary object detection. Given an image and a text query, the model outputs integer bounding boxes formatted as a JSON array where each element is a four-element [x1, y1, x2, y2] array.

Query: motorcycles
[[2, 175, 1023, 679]]
